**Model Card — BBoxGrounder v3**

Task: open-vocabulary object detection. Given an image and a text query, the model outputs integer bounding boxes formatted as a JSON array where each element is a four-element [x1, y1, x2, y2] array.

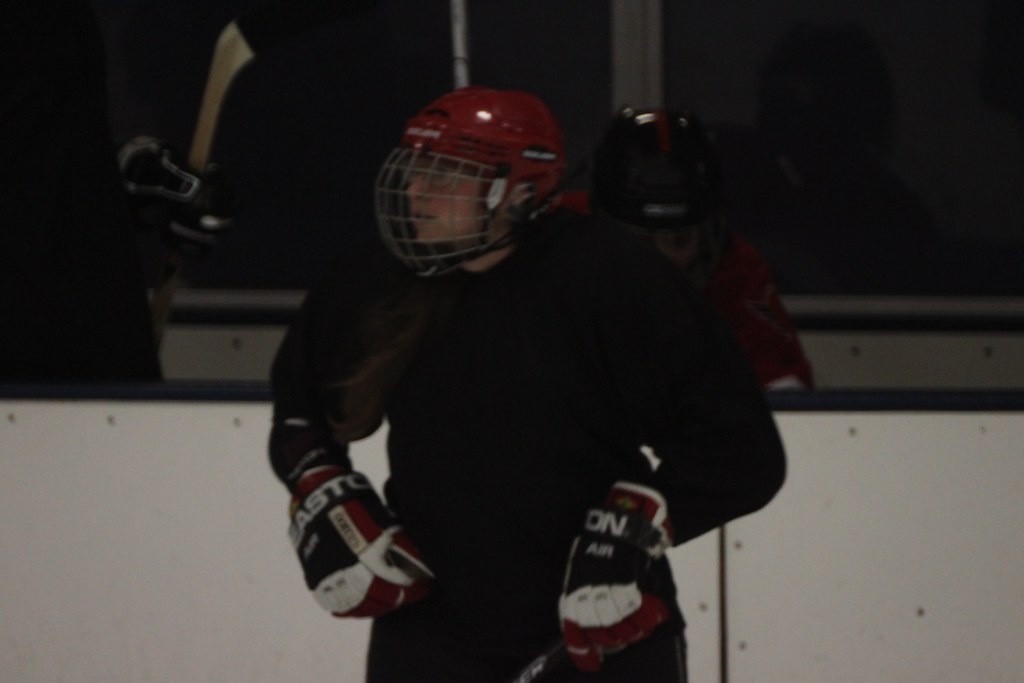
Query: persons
[[268, 85, 787, 683], [0, 1, 241, 384], [593, 101, 816, 395]]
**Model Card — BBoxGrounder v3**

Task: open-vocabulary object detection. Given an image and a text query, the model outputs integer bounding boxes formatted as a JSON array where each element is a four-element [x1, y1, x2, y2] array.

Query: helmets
[[590, 104, 729, 234], [373, 85, 566, 280]]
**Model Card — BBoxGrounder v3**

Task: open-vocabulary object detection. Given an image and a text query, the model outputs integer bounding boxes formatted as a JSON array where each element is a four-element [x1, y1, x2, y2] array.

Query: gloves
[[113, 135, 240, 262], [288, 452, 437, 620], [561, 480, 676, 669]]
